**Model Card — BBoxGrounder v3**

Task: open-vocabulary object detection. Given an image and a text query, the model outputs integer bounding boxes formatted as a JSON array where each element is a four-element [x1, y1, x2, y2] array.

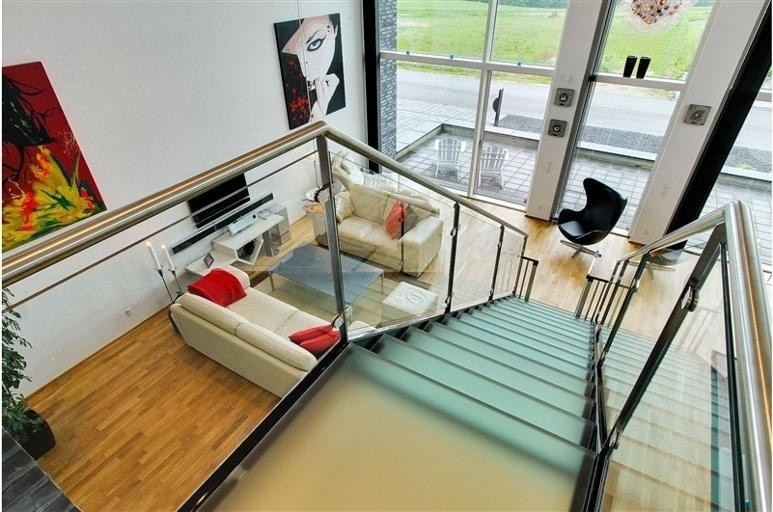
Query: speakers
[[636, 56, 651, 79], [623, 56, 637, 77]]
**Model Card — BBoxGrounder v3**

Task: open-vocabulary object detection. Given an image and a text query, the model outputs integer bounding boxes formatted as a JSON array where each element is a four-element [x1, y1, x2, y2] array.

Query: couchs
[[310, 182, 444, 278], [168, 262, 374, 399]]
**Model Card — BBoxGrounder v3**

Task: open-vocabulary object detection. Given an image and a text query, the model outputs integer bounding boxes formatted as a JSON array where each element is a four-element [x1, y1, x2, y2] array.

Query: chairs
[[477, 144, 507, 190], [556, 176, 628, 260], [330, 151, 431, 200], [434, 137, 462, 181]]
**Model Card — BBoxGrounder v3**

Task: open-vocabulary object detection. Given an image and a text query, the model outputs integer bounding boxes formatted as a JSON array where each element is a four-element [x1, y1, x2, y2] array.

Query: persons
[[275, 15, 344, 123]]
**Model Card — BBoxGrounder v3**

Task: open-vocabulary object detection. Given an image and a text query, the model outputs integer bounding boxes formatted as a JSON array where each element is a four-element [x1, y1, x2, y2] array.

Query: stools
[[379, 282, 438, 328]]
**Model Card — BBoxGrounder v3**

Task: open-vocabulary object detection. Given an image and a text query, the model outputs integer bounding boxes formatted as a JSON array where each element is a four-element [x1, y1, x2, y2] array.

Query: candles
[[144, 242, 175, 271]]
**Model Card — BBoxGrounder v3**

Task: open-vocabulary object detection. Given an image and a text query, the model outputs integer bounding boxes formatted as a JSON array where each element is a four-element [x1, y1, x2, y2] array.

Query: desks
[[184, 201, 290, 276]]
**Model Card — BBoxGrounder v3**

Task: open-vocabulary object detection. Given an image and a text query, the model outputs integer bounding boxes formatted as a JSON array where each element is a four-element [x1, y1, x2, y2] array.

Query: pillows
[[287, 323, 339, 359], [384, 201, 418, 241]]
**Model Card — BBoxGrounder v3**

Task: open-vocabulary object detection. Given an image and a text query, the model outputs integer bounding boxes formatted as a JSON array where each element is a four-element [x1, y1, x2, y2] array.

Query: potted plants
[[2, 285, 56, 462]]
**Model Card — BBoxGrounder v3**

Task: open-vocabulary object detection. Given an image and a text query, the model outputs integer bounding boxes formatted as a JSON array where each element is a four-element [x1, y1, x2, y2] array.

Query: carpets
[[249, 239, 431, 328]]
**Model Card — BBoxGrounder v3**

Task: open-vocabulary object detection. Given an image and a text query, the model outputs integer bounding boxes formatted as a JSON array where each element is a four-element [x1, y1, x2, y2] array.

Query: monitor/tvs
[[187, 173, 250, 229]]
